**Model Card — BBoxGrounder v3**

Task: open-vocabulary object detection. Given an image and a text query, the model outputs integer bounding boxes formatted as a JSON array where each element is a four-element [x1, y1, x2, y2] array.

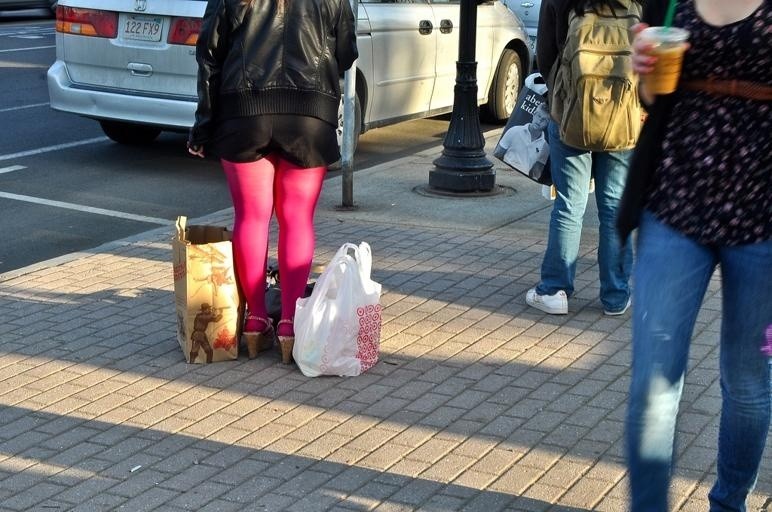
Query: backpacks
[[546, 0, 648, 153]]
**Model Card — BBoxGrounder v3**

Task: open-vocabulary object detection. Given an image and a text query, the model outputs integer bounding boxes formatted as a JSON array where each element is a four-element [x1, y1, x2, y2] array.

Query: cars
[[47, 0, 534, 171], [501, 1, 541, 70]]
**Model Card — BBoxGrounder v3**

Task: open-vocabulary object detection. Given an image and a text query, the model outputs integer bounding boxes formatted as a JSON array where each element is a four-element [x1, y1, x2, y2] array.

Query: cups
[[641, 28, 691, 94]]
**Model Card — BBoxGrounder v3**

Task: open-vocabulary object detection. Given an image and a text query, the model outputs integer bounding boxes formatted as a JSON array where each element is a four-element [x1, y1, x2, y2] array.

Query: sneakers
[[603, 298, 632, 315], [525, 288, 568, 314]]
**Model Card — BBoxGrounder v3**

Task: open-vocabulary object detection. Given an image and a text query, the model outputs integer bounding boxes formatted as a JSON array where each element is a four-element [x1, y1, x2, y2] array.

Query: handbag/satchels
[[615, 93, 674, 248], [175, 214, 240, 364], [492, 86, 594, 199]]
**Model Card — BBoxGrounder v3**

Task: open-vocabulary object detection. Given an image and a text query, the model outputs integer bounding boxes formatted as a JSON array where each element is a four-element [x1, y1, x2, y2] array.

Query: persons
[[493, 102, 551, 181], [617, 0, 772, 512], [190, 303, 222, 363], [526, 0, 650, 316], [186, 0, 358, 365]]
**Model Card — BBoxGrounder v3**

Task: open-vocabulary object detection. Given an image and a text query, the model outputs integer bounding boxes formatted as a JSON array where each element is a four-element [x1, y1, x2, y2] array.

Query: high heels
[[242, 316, 295, 364]]
